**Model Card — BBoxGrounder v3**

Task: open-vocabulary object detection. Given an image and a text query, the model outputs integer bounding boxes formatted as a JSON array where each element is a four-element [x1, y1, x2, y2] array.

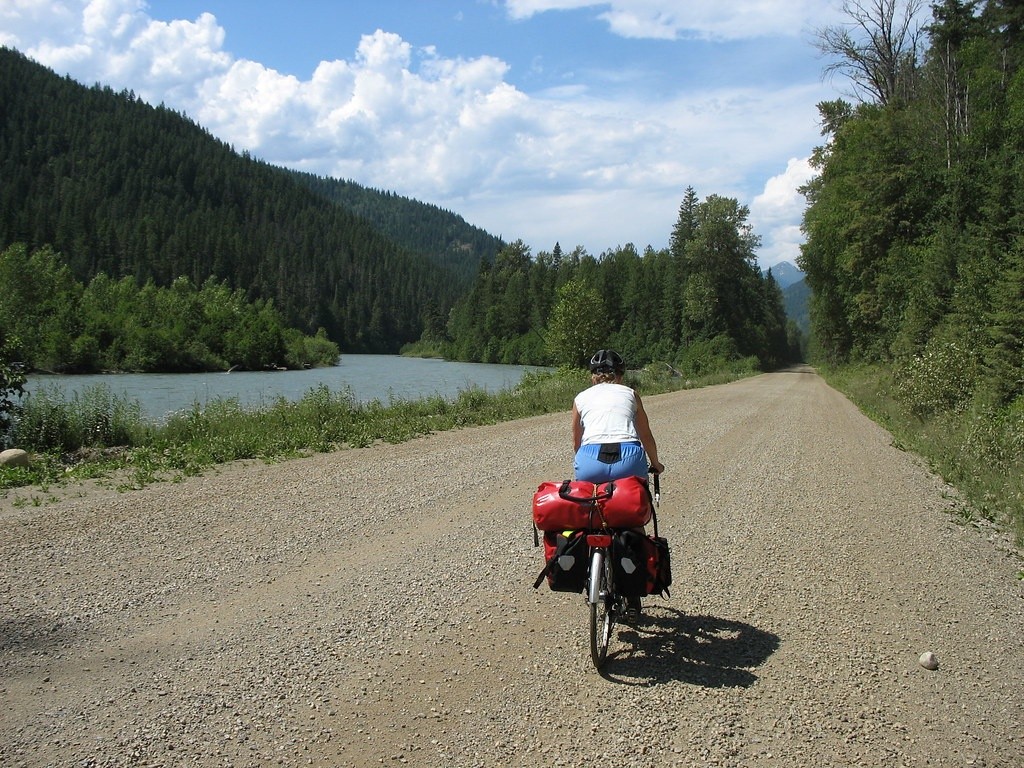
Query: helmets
[[588, 349, 625, 375]]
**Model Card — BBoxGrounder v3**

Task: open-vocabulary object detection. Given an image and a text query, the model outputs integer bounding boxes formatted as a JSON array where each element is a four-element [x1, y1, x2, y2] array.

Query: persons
[[571, 349, 665, 617]]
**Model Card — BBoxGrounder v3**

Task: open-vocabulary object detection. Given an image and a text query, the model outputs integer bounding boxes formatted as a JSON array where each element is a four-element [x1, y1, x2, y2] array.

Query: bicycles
[[583, 462, 660, 669]]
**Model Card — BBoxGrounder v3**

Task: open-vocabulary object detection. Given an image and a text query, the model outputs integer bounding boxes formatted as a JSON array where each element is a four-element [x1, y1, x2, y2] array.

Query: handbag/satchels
[[612, 530, 673, 601], [531, 476, 653, 532], [543, 528, 588, 593]]
